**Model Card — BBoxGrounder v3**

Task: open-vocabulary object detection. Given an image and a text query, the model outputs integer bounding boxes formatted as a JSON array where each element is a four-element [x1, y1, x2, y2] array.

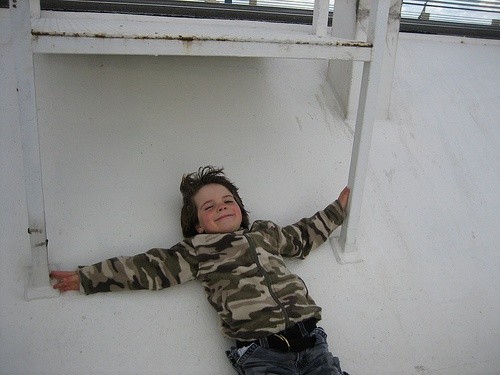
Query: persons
[[49, 164, 351, 375]]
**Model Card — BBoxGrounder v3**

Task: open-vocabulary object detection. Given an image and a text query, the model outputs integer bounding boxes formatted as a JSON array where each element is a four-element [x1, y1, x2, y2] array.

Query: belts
[[233, 316, 320, 353]]
